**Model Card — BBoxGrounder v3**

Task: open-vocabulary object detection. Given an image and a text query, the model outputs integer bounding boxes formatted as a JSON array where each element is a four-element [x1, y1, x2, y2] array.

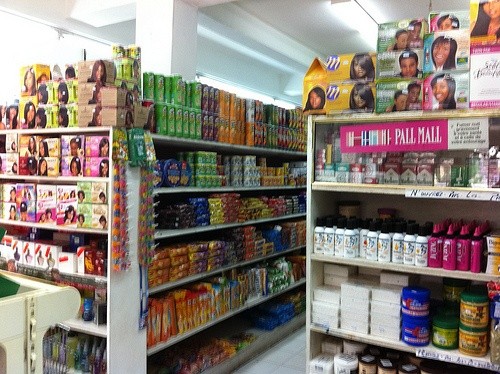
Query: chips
[[143, 72, 308, 152]]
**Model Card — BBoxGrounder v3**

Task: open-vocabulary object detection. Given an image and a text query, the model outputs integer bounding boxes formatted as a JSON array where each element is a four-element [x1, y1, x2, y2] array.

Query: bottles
[[313, 212, 491, 272]]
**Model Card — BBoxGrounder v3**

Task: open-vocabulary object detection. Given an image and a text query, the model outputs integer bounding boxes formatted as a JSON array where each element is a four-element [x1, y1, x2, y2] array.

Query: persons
[[393, 29, 412, 51], [391, 90, 408, 110], [471, 0, 500, 37], [437, 13, 460, 29], [303, 87, 327, 115], [386, 82, 423, 111], [430, 73, 457, 110], [394, 51, 425, 77], [350, 52, 376, 81], [431, 35, 457, 70], [386, 20, 424, 51], [0, 47, 158, 273], [348, 82, 376, 112]]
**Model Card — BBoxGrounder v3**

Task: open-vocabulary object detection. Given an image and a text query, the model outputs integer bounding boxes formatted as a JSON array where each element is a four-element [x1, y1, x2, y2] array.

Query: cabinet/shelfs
[[0, 125, 306, 374], [304, 105, 500, 374]]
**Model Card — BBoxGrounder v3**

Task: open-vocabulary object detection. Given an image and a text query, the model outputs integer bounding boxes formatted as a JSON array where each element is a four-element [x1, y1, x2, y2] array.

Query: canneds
[[377, 208, 396, 219], [401, 275, 489, 357], [155, 151, 307, 188], [337, 200, 363, 220], [333, 339, 441, 374]]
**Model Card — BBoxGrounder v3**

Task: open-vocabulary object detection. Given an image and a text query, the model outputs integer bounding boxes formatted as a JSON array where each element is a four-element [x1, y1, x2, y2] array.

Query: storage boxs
[[0, 59, 127, 322], [309, 263, 420, 342], [302, 0, 500, 114]]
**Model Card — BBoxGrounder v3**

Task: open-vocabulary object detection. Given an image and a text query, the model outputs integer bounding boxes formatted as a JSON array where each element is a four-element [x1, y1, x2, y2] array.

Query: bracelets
[[308, 109, 311, 115]]
